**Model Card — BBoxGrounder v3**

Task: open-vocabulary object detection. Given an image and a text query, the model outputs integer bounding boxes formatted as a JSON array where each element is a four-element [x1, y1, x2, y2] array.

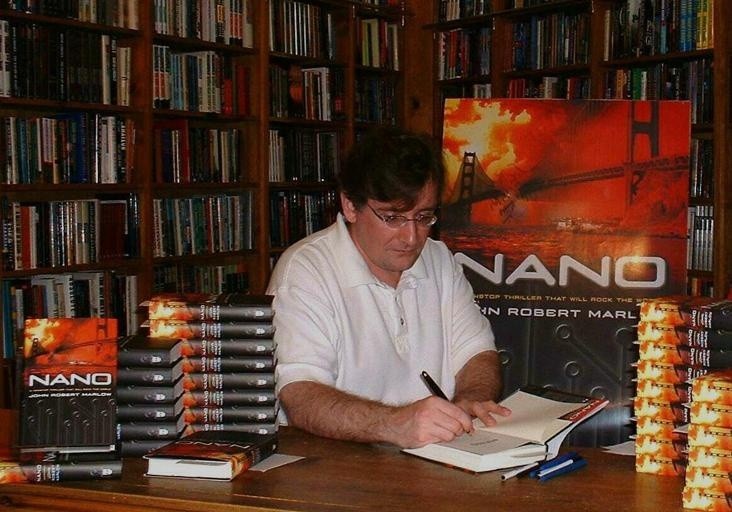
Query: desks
[[0, 425, 709, 512]]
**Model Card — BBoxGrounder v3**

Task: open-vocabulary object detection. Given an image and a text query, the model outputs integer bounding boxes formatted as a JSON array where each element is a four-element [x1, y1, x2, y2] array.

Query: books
[[155, 0, 253, 47], [603, 1, 714, 61], [630, 294, 732, 477], [400, 384, 611, 476], [153, 43, 251, 117], [686, 206, 714, 273], [685, 273, 714, 297], [151, 118, 245, 187], [602, 54, 714, 125], [270, 126, 341, 182], [437, 0, 486, 23], [502, 13, 588, 72], [0, 406, 125, 484], [149, 264, 251, 299], [4, 107, 134, 186], [506, 0, 557, 9], [434, 26, 492, 80], [13, 317, 122, 464], [360, 13, 404, 72], [270, 61, 345, 124], [270, 251, 282, 276], [2, 0, 143, 30], [113, 333, 189, 458], [269, 0, 338, 61], [682, 370, 732, 512], [0, 193, 139, 268], [1, 269, 138, 360], [141, 294, 281, 432], [505, 75, 591, 98], [689, 138, 713, 198], [356, 74, 400, 126], [268, 187, 339, 247], [360, 0, 407, 11], [0, 18, 130, 107], [436, 84, 492, 135], [149, 191, 255, 258], [141, 430, 277, 481]]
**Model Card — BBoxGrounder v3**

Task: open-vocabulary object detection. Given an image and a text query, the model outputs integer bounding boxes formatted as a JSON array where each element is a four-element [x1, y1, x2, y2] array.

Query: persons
[[266, 131, 503, 448]]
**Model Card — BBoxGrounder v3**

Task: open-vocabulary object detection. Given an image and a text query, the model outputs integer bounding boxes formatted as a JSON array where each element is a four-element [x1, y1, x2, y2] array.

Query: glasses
[[368, 205, 438, 227]]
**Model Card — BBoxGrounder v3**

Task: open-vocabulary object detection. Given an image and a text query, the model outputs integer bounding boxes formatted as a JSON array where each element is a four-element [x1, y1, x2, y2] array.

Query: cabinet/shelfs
[[150, 0, 268, 296], [266, 0, 357, 285], [0, 1, 147, 408], [500, 0, 595, 97], [358, 1, 415, 133], [415, 1, 498, 155], [594, 0, 732, 298]]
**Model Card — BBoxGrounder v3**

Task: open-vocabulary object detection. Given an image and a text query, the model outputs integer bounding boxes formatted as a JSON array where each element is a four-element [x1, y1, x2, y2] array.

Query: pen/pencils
[[422, 371, 473, 437], [537, 457, 578, 479], [516, 464, 540, 478], [500, 460, 543, 481]]
